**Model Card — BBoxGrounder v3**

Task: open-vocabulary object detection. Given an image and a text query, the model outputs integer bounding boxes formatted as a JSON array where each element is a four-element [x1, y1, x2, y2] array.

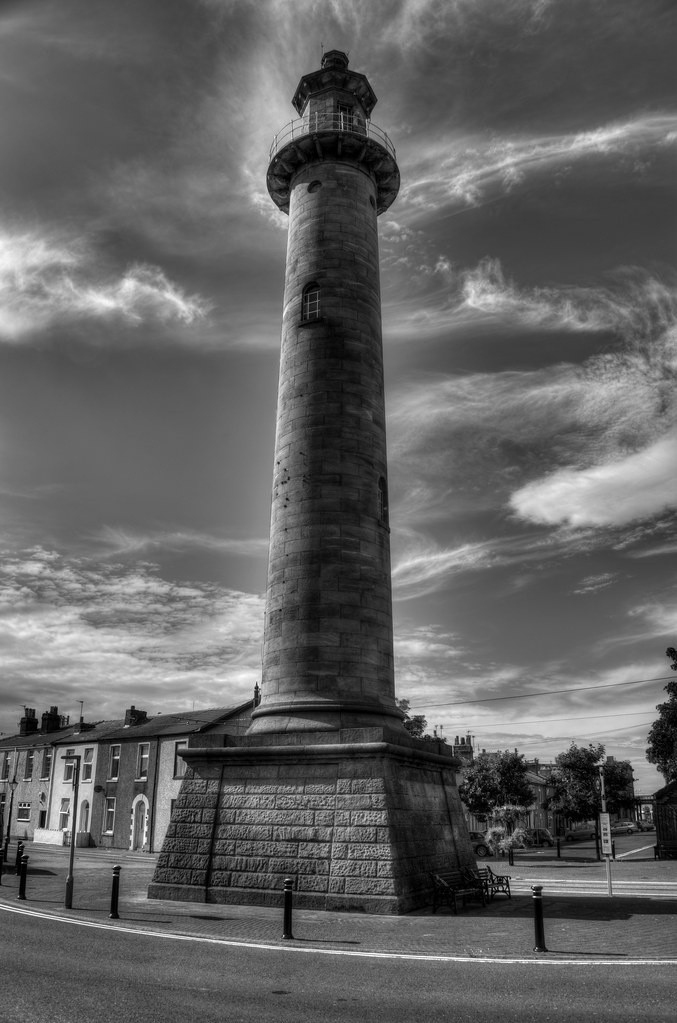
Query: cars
[[636, 820, 655, 832], [467, 829, 500, 858], [517, 828, 555, 848], [564, 824, 601, 842], [610, 821, 639, 835]]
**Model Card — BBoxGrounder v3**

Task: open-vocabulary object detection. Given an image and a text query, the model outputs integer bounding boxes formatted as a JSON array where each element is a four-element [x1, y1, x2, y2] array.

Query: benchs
[[429, 866, 487, 916], [466, 866, 510, 902], [654, 840, 677, 861]]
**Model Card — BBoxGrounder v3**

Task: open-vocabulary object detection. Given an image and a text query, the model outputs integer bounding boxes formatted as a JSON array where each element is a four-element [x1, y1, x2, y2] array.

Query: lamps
[[39, 792, 44, 802], [94, 785, 107, 797]]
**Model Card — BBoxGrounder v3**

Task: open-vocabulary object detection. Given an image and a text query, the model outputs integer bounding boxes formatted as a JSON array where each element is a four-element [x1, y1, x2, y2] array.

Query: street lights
[[60, 754, 83, 910]]
